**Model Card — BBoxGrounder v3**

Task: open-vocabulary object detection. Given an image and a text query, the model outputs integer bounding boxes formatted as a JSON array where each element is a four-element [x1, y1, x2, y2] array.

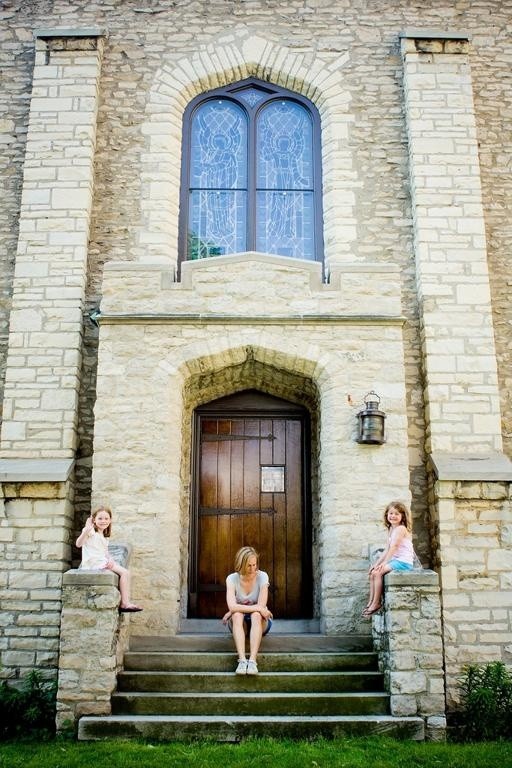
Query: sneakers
[[119, 604, 143, 612], [361, 599, 383, 617], [235, 659, 258, 675]]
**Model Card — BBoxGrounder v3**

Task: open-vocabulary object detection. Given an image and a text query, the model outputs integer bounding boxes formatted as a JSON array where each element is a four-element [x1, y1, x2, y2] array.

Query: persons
[[75, 507, 143, 613], [361, 502, 413, 615], [223, 546, 274, 675]]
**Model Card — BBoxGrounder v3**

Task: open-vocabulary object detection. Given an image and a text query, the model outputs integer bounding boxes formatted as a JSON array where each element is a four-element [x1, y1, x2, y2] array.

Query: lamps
[[356, 392, 387, 444]]
